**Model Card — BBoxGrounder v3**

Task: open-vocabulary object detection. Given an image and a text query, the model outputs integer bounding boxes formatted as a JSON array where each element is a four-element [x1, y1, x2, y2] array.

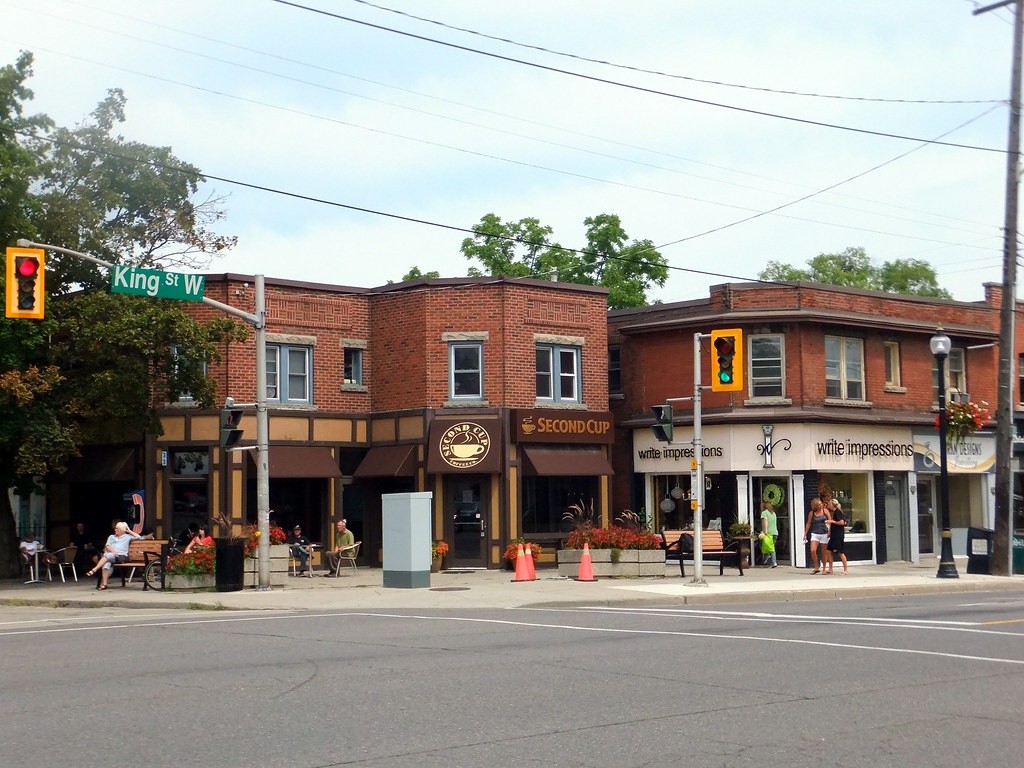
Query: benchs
[[94, 539, 169, 591], [659, 530, 744, 578]]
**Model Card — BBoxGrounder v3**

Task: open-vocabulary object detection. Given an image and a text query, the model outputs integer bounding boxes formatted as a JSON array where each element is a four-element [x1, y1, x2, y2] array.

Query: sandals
[[86, 569, 96, 578], [98, 584, 108, 591]]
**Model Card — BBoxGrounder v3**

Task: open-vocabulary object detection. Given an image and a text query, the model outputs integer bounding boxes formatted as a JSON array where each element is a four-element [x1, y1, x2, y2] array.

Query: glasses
[[199, 527, 204, 530]]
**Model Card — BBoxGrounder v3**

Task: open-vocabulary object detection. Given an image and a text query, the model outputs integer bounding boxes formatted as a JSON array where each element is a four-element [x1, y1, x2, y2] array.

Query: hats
[[294, 524, 301, 530]]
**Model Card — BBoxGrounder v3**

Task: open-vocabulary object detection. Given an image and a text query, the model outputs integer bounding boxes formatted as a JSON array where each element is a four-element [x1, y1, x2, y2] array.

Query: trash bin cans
[[1013, 534, 1024, 574], [212, 537, 249, 592], [967, 527, 995, 575]]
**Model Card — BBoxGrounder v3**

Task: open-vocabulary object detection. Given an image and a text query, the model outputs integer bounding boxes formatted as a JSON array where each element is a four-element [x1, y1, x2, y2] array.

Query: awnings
[[522, 444, 616, 476], [49, 448, 137, 481], [248, 446, 343, 478], [425, 419, 502, 473], [354, 445, 415, 477]]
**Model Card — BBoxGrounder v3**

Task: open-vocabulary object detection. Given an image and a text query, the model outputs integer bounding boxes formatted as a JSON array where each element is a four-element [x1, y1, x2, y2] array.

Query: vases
[[431, 558, 442, 573], [557, 549, 667, 577], [168, 543, 289, 588], [511, 557, 537, 572]]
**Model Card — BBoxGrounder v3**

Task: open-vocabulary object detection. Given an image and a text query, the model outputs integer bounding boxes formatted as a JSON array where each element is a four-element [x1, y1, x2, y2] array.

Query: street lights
[[928, 320, 960, 579]]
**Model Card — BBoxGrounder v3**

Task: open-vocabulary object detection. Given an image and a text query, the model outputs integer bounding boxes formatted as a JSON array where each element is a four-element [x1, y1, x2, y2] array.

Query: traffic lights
[[650, 405, 674, 441], [711, 328, 744, 393], [219, 407, 245, 449], [5, 246, 46, 321]]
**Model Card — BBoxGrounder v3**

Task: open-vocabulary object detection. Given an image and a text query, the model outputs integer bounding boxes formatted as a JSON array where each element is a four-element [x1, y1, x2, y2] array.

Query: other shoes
[[771, 564, 777, 568], [822, 570, 827, 575], [307, 554, 314, 560], [298, 572, 306, 577], [840, 572, 848, 576], [826, 570, 833, 574], [764, 556, 772, 565], [810, 569, 820, 575], [324, 572, 336, 577]]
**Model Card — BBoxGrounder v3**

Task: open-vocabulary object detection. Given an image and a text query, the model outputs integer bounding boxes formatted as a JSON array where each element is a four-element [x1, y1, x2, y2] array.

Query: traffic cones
[[509, 543, 535, 582], [573, 542, 598, 582], [524, 544, 541, 580]]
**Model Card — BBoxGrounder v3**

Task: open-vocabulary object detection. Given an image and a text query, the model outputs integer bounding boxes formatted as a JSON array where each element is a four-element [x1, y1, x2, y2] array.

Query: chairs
[[18, 544, 50, 579], [47, 546, 78, 582], [288, 544, 313, 578], [336, 541, 362, 577]]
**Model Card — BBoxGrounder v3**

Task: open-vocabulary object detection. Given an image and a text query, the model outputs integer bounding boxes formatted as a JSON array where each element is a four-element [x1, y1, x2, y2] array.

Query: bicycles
[[141, 536, 184, 591]]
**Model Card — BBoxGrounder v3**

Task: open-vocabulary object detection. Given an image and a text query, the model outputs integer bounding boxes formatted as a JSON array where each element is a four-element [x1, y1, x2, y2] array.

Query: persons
[[69, 522, 97, 565], [285, 524, 314, 576], [324, 521, 355, 578], [823, 499, 847, 574], [85, 519, 155, 590], [184, 522, 214, 553], [176, 522, 199, 550], [19, 531, 50, 582], [760, 501, 779, 568], [803, 498, 829, 575]]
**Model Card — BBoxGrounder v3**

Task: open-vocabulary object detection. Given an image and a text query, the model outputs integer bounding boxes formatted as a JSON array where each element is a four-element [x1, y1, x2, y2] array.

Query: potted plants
[[726, 512, 753, 570]]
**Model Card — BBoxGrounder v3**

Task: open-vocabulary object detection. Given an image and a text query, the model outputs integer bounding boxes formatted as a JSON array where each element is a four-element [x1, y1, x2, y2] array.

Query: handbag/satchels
[[761, 534, 774, 554]]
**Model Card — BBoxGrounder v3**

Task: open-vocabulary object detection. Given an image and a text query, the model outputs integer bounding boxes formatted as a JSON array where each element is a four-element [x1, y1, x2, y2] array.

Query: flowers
[[931, 396, 989, 448], [165, 517, 286, 576], [567, 521, 663, 550], [503, 537, 542, 560], [432, 540, 448, 560]]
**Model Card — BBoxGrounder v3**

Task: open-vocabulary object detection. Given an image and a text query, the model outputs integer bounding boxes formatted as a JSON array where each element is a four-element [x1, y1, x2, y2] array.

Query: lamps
[[659, 475, 684, 513]]
